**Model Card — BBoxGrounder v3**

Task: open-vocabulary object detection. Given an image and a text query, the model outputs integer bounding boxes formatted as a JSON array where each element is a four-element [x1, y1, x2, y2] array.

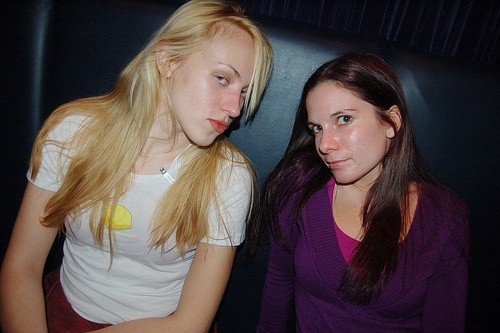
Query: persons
[[0, 0, 274, 333], [240, 48, 469, 333]]
